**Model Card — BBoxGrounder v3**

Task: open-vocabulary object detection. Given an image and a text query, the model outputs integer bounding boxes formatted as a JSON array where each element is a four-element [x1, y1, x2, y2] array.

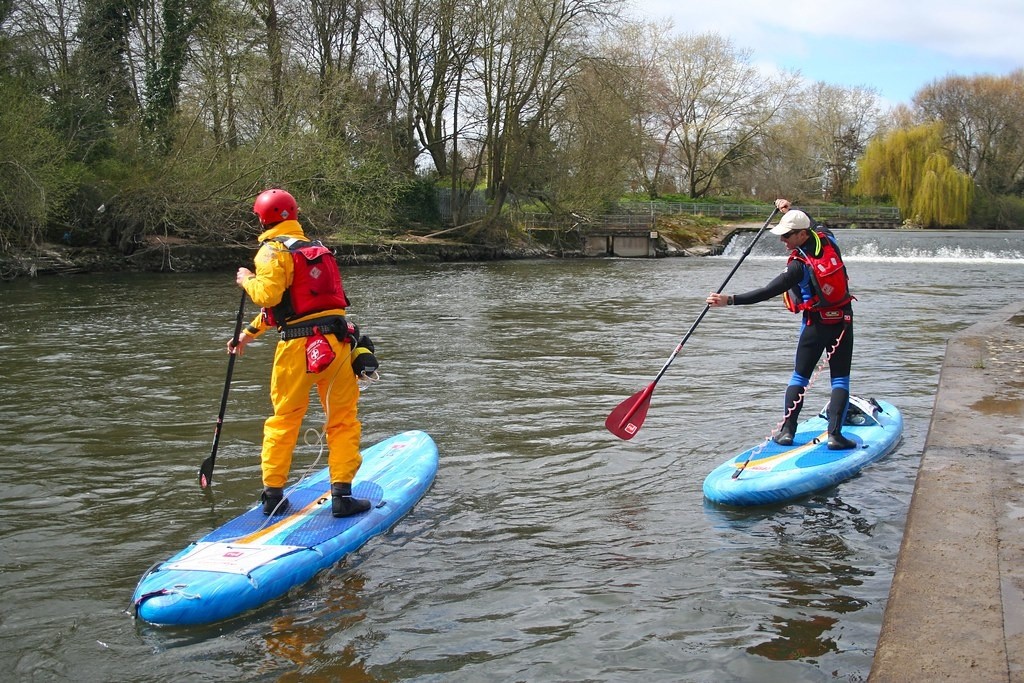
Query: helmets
[[253, 188, 298, 225]]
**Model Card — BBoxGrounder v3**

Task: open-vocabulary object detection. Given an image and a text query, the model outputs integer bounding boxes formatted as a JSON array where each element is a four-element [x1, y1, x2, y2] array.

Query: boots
[[331, 482, 371, 517], [261, 486, 289, 514]]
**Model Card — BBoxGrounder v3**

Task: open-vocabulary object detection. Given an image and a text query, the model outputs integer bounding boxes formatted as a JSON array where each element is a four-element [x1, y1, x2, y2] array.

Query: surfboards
[[130, 428, 440, 628], [702, 397, 905, 509]]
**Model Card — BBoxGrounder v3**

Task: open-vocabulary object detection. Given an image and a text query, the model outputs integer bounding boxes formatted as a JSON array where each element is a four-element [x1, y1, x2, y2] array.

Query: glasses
[[782, 232, 795, 238]]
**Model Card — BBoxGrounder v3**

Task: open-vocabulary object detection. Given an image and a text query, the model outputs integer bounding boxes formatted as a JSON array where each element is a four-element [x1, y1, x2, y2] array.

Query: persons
[[705, 198, 857, 450], [226, 188, 371, 517]]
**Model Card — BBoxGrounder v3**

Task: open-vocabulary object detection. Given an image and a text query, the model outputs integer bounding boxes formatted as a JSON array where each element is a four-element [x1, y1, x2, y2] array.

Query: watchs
[[727, 295, 733, 305]]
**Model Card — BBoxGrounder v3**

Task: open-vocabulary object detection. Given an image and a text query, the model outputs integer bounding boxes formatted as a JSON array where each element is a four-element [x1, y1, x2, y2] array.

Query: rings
[[715, 300, 718, 304]]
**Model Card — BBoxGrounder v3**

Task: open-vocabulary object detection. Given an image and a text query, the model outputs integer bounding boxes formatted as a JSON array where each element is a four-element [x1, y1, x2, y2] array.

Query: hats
[[769, 210, 810, 235]]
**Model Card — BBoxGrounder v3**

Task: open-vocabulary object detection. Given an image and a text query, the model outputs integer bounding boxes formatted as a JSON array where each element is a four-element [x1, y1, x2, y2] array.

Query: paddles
[[198, 288, 247, 490], [604, 200, 789, 441]]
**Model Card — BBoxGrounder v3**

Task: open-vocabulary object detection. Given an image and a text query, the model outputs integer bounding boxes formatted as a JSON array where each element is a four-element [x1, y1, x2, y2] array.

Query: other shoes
[[828, 434, 856, 450], [772, 432, 795, 445]]
